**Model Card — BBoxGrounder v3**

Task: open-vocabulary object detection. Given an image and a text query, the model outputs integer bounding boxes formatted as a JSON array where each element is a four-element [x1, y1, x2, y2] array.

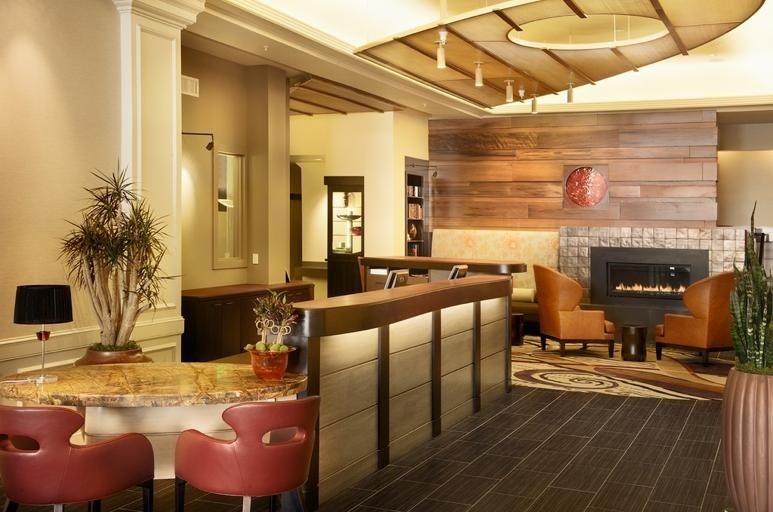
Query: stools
[[620, 323, 648, 361], [511, 313, 526, 347]]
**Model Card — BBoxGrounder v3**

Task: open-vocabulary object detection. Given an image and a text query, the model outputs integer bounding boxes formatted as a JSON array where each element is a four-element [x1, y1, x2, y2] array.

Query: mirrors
[[210, 149, 247, 270]]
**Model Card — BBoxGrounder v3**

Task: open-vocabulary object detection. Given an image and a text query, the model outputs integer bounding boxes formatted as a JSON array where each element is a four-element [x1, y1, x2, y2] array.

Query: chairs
[[0, 403, 155, 511], [172, 394, 321, 510], [654, 270, 743, 368], [530, 263, 617, 359]]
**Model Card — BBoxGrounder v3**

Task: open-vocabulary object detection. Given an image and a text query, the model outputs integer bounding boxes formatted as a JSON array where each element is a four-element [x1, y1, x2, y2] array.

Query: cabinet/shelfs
[[403, 155, 434, 275], [180, 280, 315, 362], [323, 175, 365, 261]]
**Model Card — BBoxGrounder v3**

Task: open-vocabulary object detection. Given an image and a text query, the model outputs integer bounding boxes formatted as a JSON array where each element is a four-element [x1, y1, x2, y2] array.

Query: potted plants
[[716, 198, 772, 512], [242, 287, 298, 380], [52, 156, 185, 365]]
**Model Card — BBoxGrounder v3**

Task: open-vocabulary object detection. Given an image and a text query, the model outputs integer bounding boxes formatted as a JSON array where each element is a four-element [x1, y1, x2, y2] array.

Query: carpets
[[510, 333, 735, 403]]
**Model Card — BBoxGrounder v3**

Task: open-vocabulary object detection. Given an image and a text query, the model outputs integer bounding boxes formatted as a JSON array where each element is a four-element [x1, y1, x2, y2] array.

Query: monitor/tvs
[[384, 269, 409, 290], [448, 264, 468, 280]]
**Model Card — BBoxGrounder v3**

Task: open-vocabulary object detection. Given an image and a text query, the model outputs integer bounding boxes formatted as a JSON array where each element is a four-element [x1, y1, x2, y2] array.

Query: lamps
[[10, 282, 74, 385], [434, 37, 575, 113], [182, 130, 214, 151]]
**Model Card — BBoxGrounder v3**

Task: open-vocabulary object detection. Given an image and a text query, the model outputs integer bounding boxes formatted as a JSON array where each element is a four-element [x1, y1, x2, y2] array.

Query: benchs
[[510, 299, 540, 336]]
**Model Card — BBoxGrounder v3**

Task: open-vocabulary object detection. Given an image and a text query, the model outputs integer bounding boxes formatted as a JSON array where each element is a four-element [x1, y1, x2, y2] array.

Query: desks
[[0, 360, 307, 510]]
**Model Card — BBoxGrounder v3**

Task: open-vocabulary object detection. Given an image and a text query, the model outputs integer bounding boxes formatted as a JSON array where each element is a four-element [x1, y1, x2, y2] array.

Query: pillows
[[511, 287, 535, 303]]
